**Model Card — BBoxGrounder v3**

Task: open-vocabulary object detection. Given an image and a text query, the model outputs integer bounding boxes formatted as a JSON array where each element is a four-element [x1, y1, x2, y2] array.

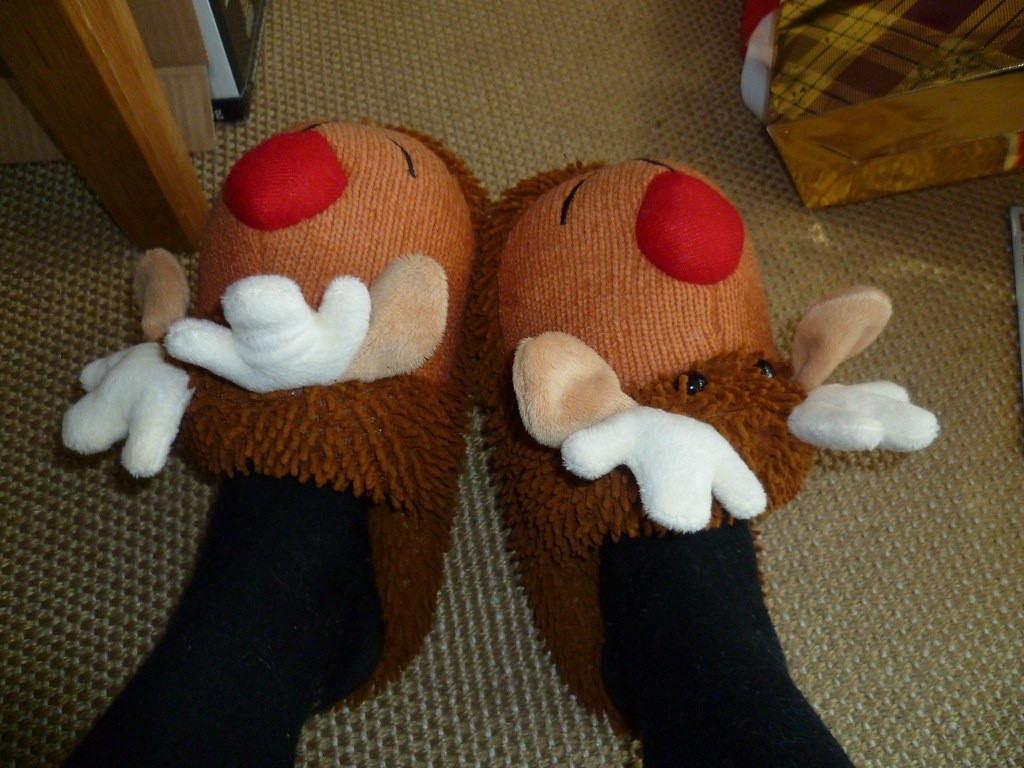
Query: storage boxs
[[0, 0, 267, 170]]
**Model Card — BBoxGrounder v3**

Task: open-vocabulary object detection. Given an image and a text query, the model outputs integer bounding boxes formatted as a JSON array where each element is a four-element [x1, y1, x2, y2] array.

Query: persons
[[43, 119, 938, 766]]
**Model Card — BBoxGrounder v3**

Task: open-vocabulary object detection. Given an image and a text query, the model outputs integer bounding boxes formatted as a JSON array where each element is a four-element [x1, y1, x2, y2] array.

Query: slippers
[[462, 154, 946, 734], [60, 114, 489, 705]]
